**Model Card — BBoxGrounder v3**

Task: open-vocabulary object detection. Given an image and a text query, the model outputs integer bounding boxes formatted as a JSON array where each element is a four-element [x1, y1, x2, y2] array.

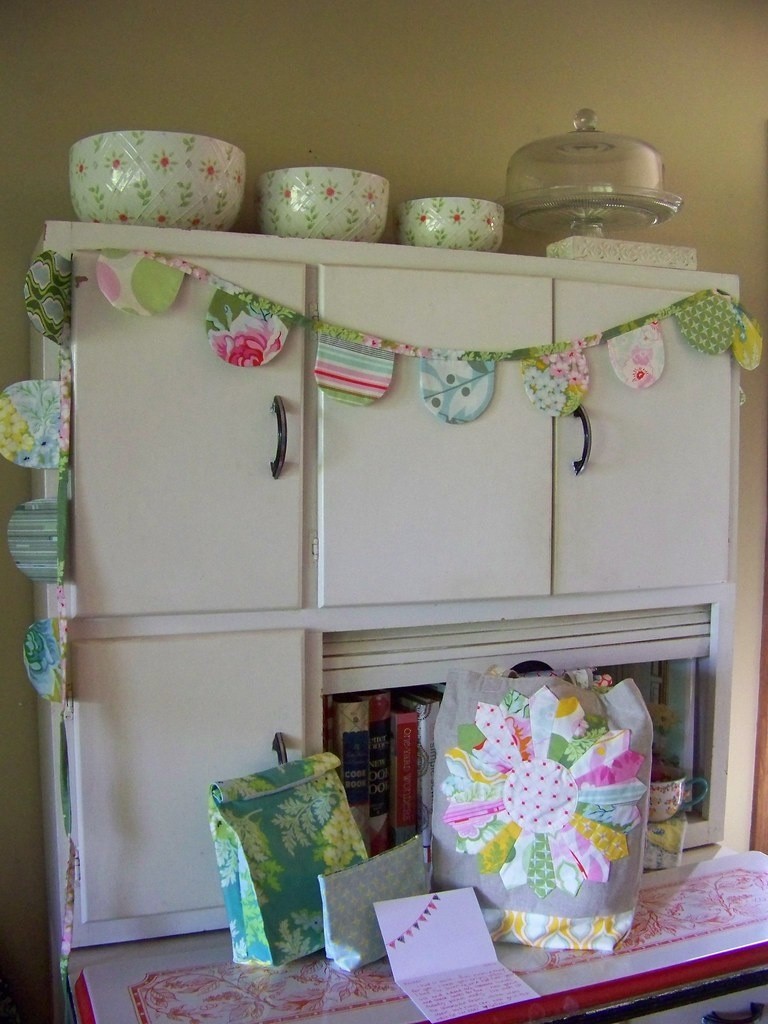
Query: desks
[[53, 842, 768, 1024]]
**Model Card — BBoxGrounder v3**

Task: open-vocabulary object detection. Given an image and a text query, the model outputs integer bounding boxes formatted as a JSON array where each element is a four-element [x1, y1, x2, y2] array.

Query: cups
[[649, 765, 708, 823]]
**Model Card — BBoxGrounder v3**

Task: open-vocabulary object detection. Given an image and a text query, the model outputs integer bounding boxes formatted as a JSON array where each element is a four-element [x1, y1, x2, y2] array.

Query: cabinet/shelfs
[[24, 222, 736, 935]]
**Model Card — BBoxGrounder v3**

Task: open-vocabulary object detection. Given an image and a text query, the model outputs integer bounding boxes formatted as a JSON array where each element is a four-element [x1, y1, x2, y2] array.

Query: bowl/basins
[[68, 130, 246, 231], [255, 166, 390, 242], [397, 197, 505, 251]]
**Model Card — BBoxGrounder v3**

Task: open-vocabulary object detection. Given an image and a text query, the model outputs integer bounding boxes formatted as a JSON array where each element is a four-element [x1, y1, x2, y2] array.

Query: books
[[362, 690, 390, 858], [334, 695, 371, 861], [389, 706, 418, 847], [399, 692, 440, 872], [323, 695, 335, 759]]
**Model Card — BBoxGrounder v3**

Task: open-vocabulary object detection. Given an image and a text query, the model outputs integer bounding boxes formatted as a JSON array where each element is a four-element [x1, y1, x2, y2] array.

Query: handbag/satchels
[[430, 667, 653, 953], [318, 835, 427, 973], [208, 752, 370, 968]]
